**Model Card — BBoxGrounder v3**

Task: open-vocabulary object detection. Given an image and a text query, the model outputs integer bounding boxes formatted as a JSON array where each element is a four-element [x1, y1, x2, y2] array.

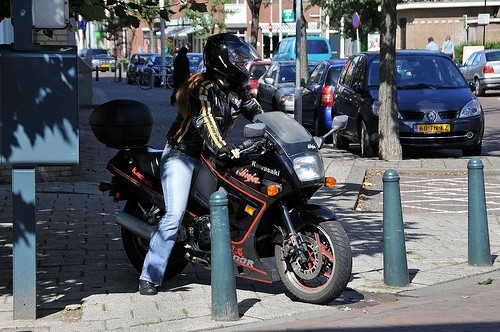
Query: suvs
[[79, 48, 118, 71]]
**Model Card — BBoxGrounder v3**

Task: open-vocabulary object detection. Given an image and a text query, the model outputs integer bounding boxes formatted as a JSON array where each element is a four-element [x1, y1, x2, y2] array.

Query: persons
[[138, 33, 267, 296], [170, 46, 190, 106], [442, 35, 455, 62], [424, 36, 440, 50]]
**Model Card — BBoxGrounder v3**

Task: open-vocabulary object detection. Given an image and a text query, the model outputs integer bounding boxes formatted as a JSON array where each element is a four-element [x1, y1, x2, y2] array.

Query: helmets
[[202, 32, 251, 88]]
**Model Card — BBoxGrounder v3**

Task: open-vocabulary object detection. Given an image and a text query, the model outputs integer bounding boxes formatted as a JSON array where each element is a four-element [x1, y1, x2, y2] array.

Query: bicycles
[[137, 62, 175, 91]]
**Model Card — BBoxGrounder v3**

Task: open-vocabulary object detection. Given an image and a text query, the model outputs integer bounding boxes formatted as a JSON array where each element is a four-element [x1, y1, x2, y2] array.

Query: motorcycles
[[87, 98, 353, 305]]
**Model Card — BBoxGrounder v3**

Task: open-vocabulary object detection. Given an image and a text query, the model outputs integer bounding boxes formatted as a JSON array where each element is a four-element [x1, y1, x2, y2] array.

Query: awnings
[[259, 21, 322, 36], [144, 26, 203, 39]]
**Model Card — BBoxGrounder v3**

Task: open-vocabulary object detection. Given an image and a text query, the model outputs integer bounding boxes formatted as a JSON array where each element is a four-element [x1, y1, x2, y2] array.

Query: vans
[[268, 35, 332, 73]]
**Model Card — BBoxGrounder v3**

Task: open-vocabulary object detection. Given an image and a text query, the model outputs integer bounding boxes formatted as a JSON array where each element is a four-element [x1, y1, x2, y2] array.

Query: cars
[[459, 49, 500, 96], [256, 60, 322, 114], [170, 52, 208, 77], [294, 59, 349, 144], [330, 48, 485, 160], [126, 53, 174, 83], [244, 59, 274, 99]]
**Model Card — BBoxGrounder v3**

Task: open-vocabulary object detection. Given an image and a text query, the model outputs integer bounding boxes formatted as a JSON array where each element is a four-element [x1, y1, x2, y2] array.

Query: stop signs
[[268, 23, 273, 32]]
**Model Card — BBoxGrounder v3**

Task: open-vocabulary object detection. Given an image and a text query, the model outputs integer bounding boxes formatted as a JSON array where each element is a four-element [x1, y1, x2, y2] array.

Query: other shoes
[[138, 279, 158, 295]]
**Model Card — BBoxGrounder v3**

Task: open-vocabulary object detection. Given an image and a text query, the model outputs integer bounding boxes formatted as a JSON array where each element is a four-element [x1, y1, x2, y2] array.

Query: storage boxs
[[89, 99, 156, 150]]
